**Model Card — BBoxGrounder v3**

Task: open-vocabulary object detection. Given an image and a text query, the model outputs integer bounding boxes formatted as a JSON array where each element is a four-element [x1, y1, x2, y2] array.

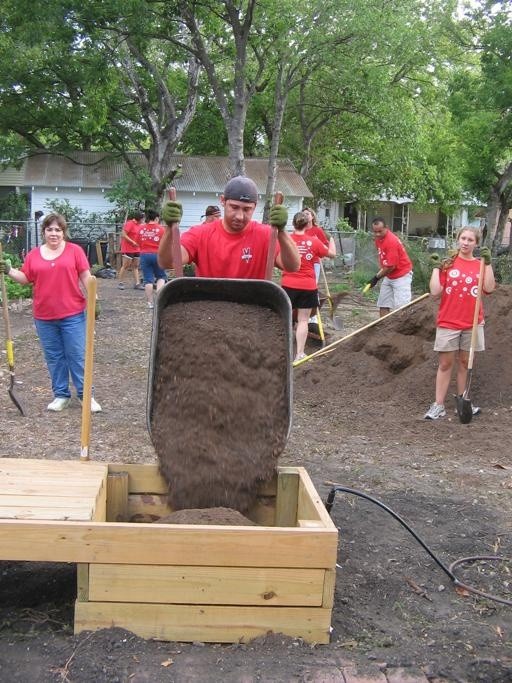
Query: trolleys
[[143, 184, 296, 478]]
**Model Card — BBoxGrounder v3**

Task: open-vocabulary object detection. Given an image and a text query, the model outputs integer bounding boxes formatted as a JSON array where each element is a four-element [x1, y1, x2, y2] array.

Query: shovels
[[349, 282, 373, 306], [453, 256, 485, 424], [0, 243, 28, 417], [320, 261, 343, 331]]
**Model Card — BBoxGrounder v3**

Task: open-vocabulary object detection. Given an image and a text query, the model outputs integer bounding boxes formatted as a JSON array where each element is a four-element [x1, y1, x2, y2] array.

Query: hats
[[224, 176, 257, 203], [202, 206, 221, 217]]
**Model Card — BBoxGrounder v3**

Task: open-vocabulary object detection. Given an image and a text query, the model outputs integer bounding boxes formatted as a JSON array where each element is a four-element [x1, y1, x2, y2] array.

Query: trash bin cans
[[88, 241, 108, 267], [70, 237, 89, 257]]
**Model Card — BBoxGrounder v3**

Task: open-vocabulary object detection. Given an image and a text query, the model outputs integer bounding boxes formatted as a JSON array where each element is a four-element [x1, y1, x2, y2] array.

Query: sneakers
[[48, 397, 72, 412], [424, 402, 446, 419], [294, 353, 307, 361], [78, 397, 102, 412], [455, 402, 481, 415], [118, 283, 145, 290]]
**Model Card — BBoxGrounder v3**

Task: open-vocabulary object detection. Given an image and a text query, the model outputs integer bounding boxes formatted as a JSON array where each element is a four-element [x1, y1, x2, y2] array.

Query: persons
[[200, 205, 222, 225], [300, 207, 330, 316], [119, 210, 145, 289], [420, 225, 496, 423], [136, 209, 167, 309], [1, 213, 103, 415], [364, 218, 415, 324], [157, 174, 302, 280], [281, 212, 336, 361]]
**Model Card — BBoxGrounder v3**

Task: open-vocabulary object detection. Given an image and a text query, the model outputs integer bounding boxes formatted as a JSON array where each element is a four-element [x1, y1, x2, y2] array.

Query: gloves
[[0, 260, 10, 274], [430, 253, 441, 269], [366, 276, 379, 288], [269, 204, 288, 231], [94, 299, 100, 320], [480, 247, 491, 264], [163, 200, 182, 226]]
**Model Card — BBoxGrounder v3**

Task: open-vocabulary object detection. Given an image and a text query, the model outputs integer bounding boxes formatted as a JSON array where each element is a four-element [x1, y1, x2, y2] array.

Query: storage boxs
[[0, 453, 341, 647]]
